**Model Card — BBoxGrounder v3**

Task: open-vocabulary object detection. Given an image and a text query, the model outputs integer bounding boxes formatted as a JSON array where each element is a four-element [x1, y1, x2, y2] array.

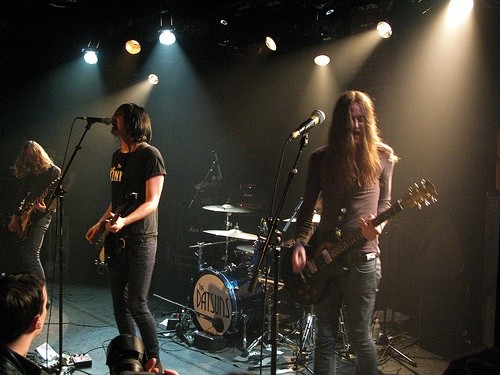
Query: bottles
[[460, 331, 470, 344], [371, 318, 380, 340]]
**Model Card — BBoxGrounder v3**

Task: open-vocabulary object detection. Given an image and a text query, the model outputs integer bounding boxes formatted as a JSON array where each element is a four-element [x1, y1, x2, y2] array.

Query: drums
[[233, 246, 281, 279], [191, 268, 271, 338]]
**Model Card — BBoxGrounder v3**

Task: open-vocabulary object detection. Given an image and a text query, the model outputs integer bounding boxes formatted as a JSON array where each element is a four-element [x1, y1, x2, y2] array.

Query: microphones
[[79, 117, 111, 125], [214, 152, 222, 179], [288, 110, 326, 142]]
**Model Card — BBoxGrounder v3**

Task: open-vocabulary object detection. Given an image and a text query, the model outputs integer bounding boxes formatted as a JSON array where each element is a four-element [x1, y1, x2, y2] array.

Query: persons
[[292, 91, 397, 375], [8, 140, 63, 284], [0, 275, 176, 375], [86, 103, 167, 372]]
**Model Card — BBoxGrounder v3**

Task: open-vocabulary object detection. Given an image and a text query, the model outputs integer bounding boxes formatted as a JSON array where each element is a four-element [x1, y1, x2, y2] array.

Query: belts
[[320, 229, 354, 243], [108, 237, 150, 250]]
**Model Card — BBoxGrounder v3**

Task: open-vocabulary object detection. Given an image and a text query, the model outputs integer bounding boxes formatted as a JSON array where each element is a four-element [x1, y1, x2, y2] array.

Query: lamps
[[313, 6, 338, 44], [82, 39, 104, 64], [251, 36, 277, 60], [158, 11, 177, 47]]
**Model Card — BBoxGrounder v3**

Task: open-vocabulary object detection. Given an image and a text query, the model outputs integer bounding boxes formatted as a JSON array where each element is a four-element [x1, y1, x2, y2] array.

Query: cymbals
[[203, 228, 267, 241], [202, 204, 255, 213]]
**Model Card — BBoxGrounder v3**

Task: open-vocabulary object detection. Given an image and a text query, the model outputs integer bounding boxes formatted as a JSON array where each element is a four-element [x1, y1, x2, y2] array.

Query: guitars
[[282, 179, 438, 304], [15, 169, 63, 242], [89, 191, 140, 266]]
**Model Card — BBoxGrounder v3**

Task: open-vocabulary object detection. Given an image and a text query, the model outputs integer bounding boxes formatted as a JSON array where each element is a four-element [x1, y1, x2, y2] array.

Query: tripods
[[243, 279, 315, 375], [152, 293, 216, 347], [373, 221, 420, 369]]
